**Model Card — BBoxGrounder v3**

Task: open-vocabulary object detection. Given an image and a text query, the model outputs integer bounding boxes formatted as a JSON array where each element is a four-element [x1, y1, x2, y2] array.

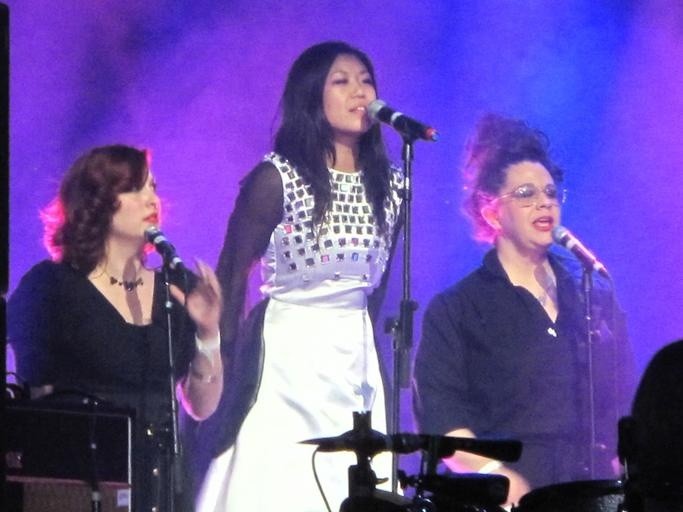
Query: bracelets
[[476, 457, 503, 474], [186, 367, 223, 386], [192, 332, 226, 357]]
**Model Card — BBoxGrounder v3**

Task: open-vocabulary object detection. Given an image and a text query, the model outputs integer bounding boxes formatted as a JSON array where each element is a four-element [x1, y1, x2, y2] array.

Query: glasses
[[492, 185, 567, 206]]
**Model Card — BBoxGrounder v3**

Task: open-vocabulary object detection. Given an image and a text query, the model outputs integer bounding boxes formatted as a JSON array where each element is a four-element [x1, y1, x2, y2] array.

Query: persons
[[614, 325, 681, 509], [407, 106, 631, 510], [188, 41, 413, 509], [4, 138, 224, 510]]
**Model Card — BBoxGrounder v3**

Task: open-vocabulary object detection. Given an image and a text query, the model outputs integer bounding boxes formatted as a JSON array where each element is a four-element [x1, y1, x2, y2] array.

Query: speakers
[[4, 404, 136, 512]]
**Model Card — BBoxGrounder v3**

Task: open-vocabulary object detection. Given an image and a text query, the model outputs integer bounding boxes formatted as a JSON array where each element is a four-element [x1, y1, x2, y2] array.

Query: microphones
[[368, 100, 441, 143], [551, 226, 611, 279], [144, 226, 187, 274]]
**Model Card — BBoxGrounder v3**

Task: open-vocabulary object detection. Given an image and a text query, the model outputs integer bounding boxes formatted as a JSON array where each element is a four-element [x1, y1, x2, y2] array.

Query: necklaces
[[98, 262, 147, 293]]
